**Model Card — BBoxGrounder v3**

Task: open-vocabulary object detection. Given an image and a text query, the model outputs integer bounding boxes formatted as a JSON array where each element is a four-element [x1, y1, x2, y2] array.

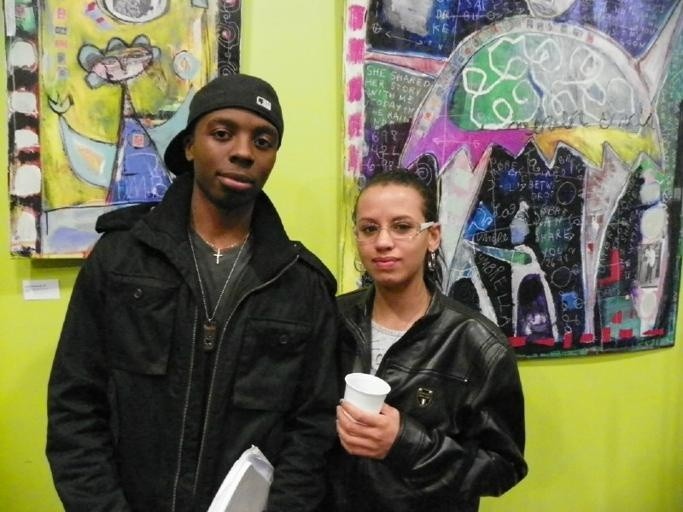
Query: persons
[[332, 170, 530, 512], [46, 71, 339, 510]]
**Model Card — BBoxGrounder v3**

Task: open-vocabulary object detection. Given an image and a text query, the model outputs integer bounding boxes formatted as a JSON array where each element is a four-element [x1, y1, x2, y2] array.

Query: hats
[[163, 73, 283, 177]]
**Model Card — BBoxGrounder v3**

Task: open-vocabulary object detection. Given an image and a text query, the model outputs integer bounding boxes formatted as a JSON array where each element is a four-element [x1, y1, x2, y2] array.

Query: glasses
[[353, 220, 433, 241]]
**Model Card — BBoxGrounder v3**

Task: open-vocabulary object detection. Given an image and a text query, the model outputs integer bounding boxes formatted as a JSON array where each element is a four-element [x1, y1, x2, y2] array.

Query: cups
[[342, 372, 393, 417]]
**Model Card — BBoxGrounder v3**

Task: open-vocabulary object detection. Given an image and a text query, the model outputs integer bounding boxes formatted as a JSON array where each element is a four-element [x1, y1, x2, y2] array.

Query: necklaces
[[186, 232, 251, 353], [186, 221, 251, 264]]
